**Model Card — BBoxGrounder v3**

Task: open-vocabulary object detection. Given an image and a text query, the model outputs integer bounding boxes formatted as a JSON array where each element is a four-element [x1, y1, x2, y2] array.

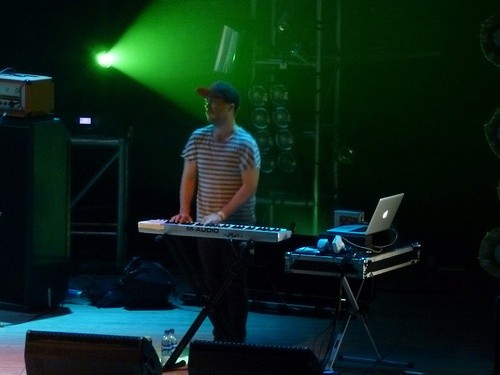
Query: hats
[[197, 81, 240, 106]]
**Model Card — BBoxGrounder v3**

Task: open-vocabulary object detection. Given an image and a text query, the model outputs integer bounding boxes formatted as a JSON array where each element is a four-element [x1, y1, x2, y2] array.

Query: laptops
[[327, 193, 404, 235]]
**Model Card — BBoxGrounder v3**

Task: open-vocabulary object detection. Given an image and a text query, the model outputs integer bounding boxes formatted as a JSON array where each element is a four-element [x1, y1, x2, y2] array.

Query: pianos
[[138, 218, 292, 243]]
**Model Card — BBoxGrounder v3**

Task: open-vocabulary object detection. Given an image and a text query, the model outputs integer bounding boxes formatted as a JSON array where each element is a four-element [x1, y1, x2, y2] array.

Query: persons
[[170, 81, 262, 344]]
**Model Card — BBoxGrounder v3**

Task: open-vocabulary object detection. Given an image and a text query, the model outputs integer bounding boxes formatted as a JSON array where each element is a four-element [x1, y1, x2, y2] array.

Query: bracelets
[[217, 211, 227, 221]]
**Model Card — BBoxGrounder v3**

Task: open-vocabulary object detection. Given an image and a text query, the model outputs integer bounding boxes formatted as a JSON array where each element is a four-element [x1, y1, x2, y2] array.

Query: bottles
[[168, 329, 177, 356], [161, 331, 171, 367]]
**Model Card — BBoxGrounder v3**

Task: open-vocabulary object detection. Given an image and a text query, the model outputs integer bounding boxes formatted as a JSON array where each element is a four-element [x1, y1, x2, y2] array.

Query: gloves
[[201, 212, 222, 227]]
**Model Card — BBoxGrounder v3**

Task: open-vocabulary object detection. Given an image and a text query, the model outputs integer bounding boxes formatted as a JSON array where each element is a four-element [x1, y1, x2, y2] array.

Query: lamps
[[247, 84, 296, 174]]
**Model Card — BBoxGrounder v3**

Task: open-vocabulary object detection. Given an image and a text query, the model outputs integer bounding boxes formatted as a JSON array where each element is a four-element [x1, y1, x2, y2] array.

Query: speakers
[[24, 331, 162, 375], [188, 339, 324, 375], [0, 119, 72, 315]]
